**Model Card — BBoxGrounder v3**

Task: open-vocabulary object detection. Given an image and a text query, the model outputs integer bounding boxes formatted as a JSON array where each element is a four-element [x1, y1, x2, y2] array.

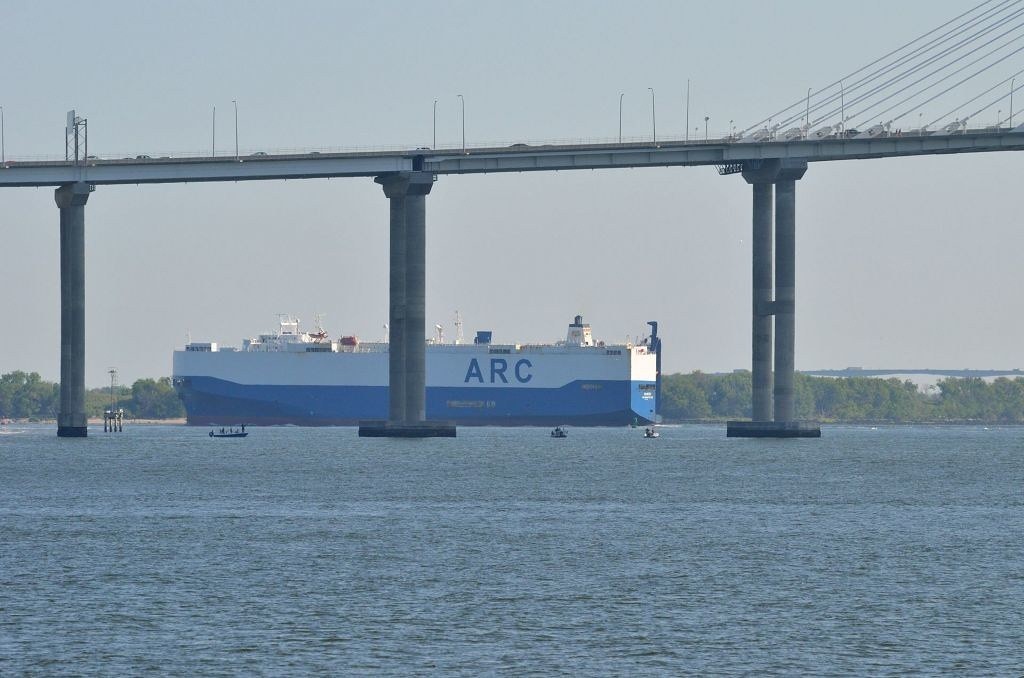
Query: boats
[[213, 432, 249, 437], [644, 425, 660, 438], [550, 426, 569, 438], [339, 335, 359, 345]]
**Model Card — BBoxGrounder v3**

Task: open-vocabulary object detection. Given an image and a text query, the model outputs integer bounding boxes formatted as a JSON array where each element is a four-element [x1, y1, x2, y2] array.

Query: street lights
[[997, 110, 1002, 132], [647, 88, 657, 148], [807, 87, 812, 140], [838, 80, 845, 138], [918, 113, 923, 136], [0, 106, 5, 163], [1009, 78, 1017, 131], [768, 118, 772, 142], [457, 94, 465, 153], [800, 118, 805, 141], [729, 119, 733, 139], [619, 94, 624, 143], [705, 116, 710, 144], [432, 99, 438, 150], [231, 100, 239, 160]]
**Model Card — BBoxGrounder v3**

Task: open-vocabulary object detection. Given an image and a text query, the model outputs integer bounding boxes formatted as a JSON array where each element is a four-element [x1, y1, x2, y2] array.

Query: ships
[[171, 306, 662, 429]]
